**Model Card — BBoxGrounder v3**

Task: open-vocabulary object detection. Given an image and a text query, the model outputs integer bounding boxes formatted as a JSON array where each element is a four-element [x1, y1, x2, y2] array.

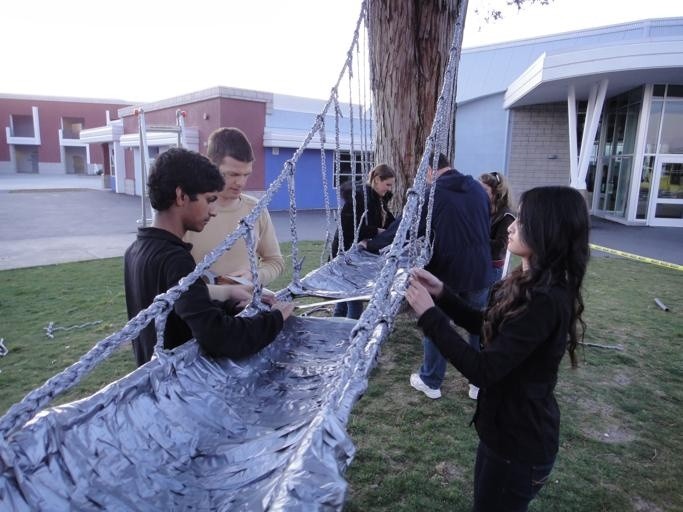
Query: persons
[[470, 171, 517, 299], [400, 185, 589, 512], [124, 148, 301, 365], [325, 164, 394, 319], [356, 151, 505, 400], [182, 128, 284, 306]]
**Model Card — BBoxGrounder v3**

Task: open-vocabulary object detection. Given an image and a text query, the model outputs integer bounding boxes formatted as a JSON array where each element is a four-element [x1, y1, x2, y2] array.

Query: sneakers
[[410, 373, 442, 399], [468, 382, 480, 399]]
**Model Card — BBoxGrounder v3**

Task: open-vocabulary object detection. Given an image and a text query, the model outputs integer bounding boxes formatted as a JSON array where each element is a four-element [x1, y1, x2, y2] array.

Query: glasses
[[490, 171, 500, 183]]
